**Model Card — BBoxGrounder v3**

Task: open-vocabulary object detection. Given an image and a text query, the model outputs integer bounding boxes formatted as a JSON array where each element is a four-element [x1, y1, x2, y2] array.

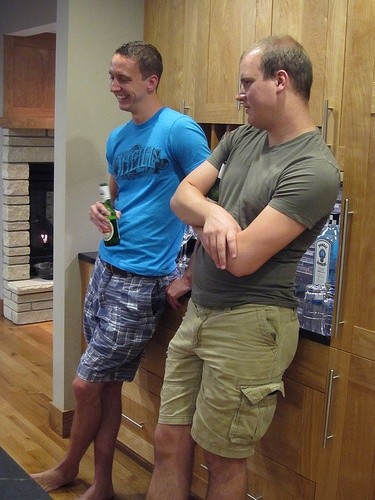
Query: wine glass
[[175, 223, 194, 270]]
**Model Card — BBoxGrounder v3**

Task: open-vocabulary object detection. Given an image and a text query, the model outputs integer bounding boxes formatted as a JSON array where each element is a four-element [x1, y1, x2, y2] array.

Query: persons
[[33, 39, 209, 499], [145, 34, 338, 499]]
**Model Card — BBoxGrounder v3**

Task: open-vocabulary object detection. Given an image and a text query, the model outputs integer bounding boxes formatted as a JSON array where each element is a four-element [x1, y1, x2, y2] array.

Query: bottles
[[294, 203, 340, 308], [99, 182, 120, 247]]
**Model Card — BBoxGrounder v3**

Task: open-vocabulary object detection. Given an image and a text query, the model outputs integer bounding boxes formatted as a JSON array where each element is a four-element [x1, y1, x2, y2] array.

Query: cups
[[301, 284, 335, 336]]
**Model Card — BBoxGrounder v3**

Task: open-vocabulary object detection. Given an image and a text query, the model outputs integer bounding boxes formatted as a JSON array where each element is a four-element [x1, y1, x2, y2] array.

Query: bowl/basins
[[32, 262, 53, 279]]
